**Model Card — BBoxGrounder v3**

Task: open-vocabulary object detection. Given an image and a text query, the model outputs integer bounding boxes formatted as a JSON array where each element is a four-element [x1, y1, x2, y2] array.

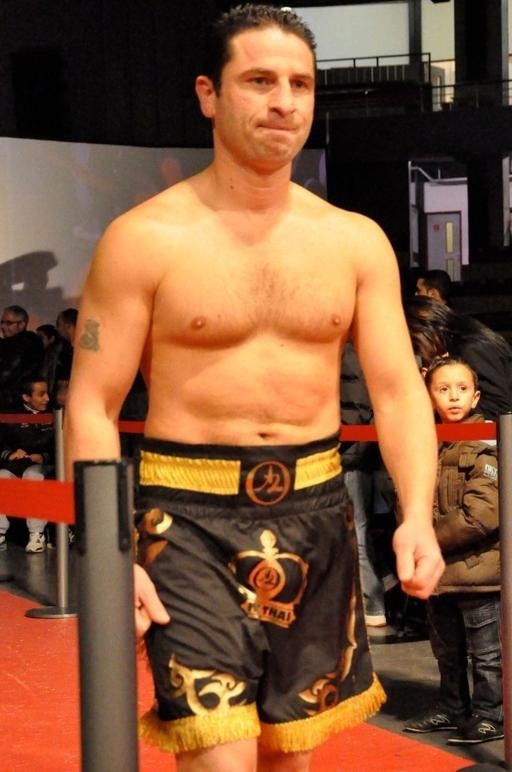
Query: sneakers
[[403, 706, 464, 733], [0, 532, 8, 545], [361, 573, 432, 643], [446, 714, 505, 746], [24, 533, 46, 553], [47, 528, 76, 549]]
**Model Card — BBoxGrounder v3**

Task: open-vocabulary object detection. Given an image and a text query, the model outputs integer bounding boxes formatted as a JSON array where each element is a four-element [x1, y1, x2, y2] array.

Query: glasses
[[0, 318, 23, 325]]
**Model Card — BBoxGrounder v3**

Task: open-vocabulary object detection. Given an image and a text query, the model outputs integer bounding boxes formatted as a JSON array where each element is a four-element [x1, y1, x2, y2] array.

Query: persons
[[400, 353, 503, 746], [1, 266, 507, 648], [63, 0, 452, 772]]
